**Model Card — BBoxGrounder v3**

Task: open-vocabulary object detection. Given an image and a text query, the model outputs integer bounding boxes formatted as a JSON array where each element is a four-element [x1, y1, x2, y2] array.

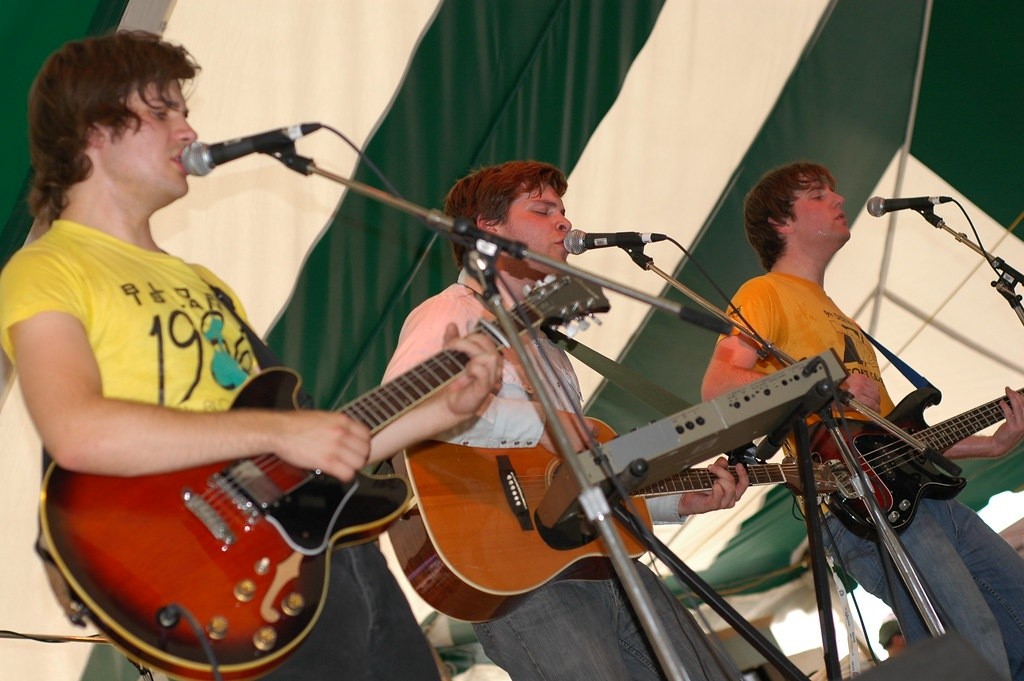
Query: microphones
[[866, 196, 952, 218], [562, 229, 667, 256], [180, 121, 323, 178]]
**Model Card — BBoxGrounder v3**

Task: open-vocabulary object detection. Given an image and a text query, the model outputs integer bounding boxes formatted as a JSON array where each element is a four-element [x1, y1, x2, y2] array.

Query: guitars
[[383, 402, 853, 624], [35, 271, 611, 681], [807, 385, 1024, 541]]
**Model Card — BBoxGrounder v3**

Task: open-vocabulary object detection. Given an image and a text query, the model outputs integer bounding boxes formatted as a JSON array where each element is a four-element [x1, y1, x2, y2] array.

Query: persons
[[702, 159, 1022, 681], [383, 157, 752, 680], [0, 19, 500, 680]]
